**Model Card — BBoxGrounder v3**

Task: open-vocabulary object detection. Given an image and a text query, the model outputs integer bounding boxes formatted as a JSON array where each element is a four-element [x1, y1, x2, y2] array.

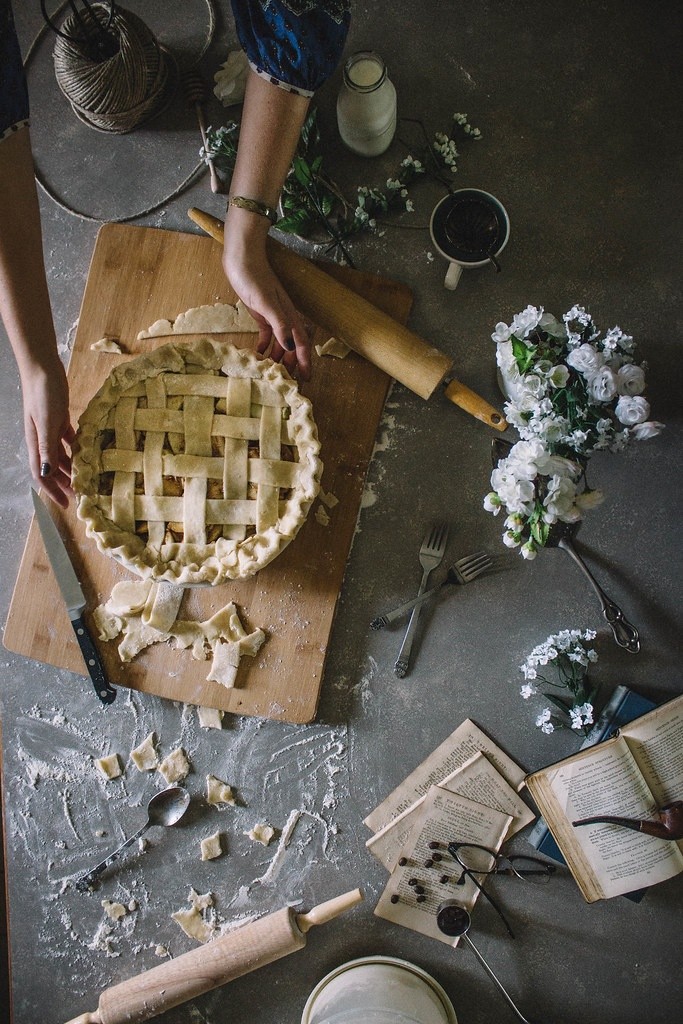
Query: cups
[[429, 189, 511, 291]]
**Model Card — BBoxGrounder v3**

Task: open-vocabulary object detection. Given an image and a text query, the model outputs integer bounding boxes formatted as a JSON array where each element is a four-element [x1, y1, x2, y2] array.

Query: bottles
[[336, 49, 394, 159]]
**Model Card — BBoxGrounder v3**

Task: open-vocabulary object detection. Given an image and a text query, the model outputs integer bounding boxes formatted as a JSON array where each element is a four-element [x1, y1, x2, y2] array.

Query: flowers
[[481, 304, 666, 559], [518, 627, 599, 739], [323, 112, 483, 256], [198, 118, 360, 271]]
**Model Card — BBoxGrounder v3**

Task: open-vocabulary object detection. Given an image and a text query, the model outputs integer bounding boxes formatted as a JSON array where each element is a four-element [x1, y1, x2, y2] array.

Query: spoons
[[75, 787, 190, 891]]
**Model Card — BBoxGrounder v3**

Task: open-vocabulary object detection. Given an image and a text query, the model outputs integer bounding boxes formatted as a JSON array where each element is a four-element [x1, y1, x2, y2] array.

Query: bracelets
[[225, 196, 277, 225]]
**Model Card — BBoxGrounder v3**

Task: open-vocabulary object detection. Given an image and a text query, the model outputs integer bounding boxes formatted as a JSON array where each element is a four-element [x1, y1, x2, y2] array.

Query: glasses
[[448, 841, 556, 940]]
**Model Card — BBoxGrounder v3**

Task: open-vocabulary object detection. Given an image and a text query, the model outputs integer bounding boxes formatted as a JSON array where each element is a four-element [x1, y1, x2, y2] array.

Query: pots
[[300, 955, 459, 1024]]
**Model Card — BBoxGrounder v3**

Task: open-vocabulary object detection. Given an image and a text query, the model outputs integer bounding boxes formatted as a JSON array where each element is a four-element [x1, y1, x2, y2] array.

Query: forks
[[393, 522, 449, 679], [368, 550, 494, 631]]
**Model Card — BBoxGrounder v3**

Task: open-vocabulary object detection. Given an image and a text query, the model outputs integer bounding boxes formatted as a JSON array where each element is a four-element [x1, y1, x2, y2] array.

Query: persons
[[0, 0, 352, 510]]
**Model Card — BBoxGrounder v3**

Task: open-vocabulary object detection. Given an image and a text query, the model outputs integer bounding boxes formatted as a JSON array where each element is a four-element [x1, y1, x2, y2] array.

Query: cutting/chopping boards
[[2, 225, 414, 724]]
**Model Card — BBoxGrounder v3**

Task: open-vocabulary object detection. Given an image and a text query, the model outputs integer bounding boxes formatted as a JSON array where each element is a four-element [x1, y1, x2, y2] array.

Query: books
[[524, 694, 683, 906], [527, 684, 659, 904]]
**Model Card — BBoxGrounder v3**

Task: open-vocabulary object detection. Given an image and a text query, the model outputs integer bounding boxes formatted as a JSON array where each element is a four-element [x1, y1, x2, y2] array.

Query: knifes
[[31, 487, 117, 704]]
